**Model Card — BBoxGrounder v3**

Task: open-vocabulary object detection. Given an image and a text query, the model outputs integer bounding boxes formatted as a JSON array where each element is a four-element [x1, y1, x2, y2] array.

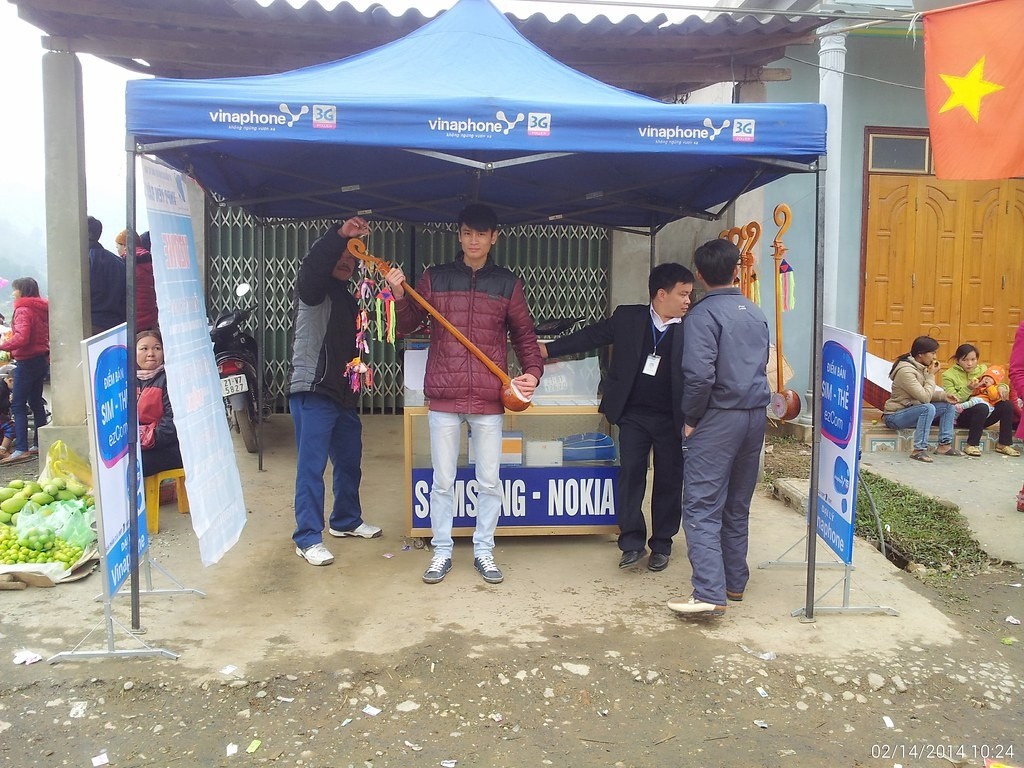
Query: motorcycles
[[207, 283, 278, 453]]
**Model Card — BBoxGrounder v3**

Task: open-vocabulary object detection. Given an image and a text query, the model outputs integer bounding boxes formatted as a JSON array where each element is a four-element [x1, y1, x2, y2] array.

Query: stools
[[144, 468, 189, 531]]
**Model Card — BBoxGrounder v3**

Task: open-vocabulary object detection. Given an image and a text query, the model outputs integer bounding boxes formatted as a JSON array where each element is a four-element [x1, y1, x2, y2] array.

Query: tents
[[123, 0, 828, 630]]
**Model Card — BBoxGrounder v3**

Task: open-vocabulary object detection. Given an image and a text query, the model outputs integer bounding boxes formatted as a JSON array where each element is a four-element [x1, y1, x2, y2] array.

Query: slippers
[[910, 452, 933, 462], [963, 446, 981, 455], [933, 448, 961, 456], [996, 445, 1020, 456]]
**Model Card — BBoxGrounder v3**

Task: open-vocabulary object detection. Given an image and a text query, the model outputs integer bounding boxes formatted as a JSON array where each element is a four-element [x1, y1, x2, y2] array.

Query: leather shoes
[[648, 552, 669, 571], [619, 548, 647, 569]]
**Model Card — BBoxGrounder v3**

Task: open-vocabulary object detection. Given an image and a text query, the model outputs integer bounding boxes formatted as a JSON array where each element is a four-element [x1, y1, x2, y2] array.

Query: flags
[[918, 0, 1024, 181]]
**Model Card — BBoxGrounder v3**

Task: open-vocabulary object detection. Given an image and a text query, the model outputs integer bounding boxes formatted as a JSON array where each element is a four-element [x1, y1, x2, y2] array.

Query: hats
[[979, 365, 1005, 384], [115, 229, 142, 247], [139, 232, 151, 252]]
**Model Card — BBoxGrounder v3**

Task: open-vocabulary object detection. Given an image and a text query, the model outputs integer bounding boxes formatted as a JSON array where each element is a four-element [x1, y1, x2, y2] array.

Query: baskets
[[159, 480, 177, 505]]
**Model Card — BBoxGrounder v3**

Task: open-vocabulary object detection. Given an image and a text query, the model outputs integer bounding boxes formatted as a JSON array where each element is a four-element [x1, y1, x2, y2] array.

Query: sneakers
[[473, 555, 504, 583], [329, 523, 382, 539], [296, 543, 334, 566], [726, 592, 743, 601], [422, 557, 452, 585], [667, 593, 726, 615]]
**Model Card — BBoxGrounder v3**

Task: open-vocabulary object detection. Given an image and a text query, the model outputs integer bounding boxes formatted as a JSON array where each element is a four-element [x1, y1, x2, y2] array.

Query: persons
[[290, 216, 383, 567], [0, 216, 183, 478], [384, 203, 544, 583], [537, 262, 695, 572], [666, 237, 770, 617], [884, 319, 1024, 511]]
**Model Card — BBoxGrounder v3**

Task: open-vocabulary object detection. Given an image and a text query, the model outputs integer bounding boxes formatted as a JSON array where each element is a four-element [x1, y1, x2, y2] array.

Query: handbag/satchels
[[139, 423, 156, 450]]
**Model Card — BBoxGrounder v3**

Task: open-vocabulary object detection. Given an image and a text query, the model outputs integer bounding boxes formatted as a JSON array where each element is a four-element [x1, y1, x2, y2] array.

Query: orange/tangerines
[[0, 525, 83, 573]]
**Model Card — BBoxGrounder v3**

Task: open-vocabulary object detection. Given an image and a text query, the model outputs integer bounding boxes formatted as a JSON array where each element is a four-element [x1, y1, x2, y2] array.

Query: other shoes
[[1016, 491, 1024, 512], [0, 446, 11, 458], [29, 448, 38, 454], [0, 451, 32, 468]]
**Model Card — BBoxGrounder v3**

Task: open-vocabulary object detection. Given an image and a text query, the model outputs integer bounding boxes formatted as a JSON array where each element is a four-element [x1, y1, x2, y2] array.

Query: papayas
[[0, 478, 87, 525]]
